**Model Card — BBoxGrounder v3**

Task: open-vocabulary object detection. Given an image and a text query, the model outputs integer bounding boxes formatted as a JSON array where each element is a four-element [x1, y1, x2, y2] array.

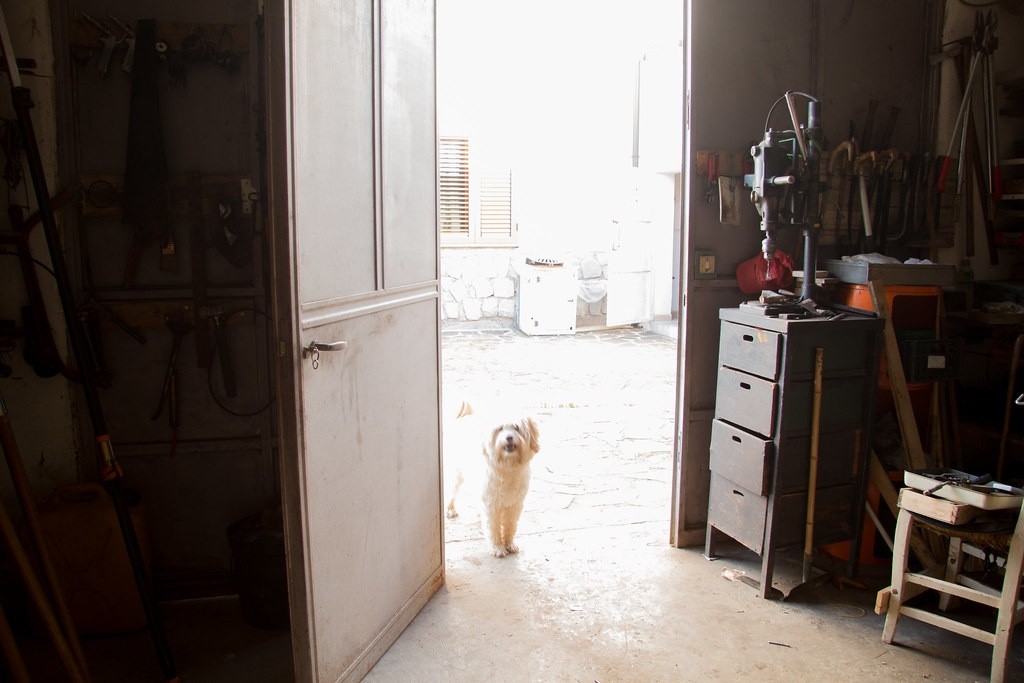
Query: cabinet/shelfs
[[701, 298, 886, 599], [814, 282, 943, 567]]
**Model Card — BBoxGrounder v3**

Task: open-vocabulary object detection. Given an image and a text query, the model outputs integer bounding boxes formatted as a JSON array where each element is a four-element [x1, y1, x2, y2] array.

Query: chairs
[[874, 487, 1024, 683]]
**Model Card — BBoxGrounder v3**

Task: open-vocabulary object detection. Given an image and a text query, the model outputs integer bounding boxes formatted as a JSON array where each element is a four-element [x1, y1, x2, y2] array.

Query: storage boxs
[[896, 327, 964, 380], [821, 259, 955, 285]]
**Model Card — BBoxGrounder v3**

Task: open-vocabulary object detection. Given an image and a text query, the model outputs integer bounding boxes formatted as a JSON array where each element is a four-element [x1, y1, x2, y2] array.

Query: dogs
[[444, 399, 540, 559]]
